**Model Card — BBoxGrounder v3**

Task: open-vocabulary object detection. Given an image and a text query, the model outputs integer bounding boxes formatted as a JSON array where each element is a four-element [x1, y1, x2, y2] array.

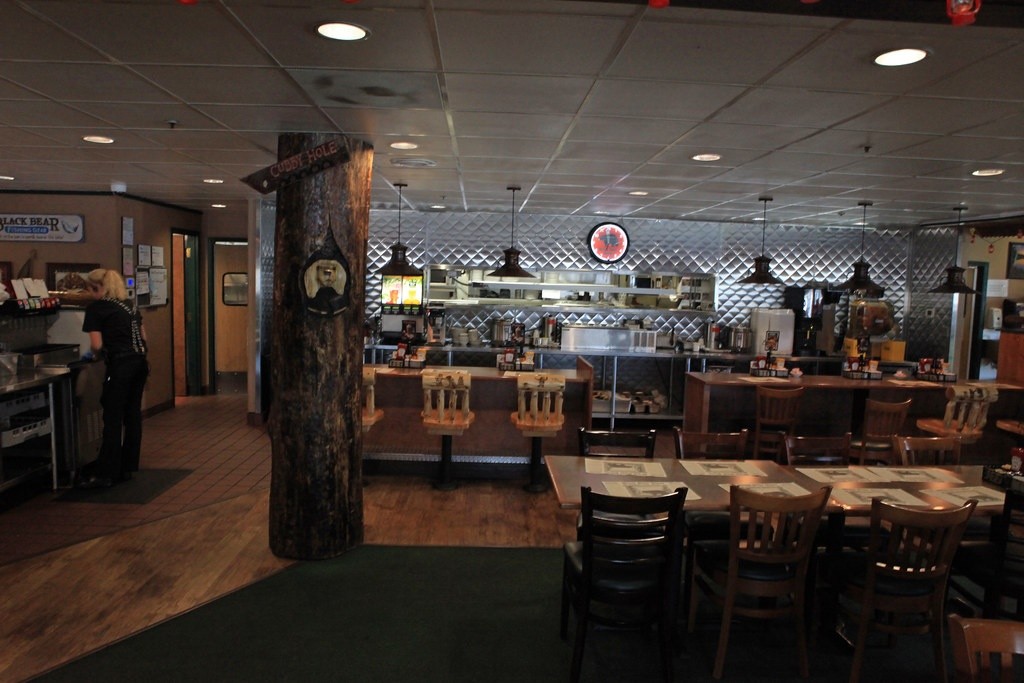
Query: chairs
[[559, 385, 1024, 683]]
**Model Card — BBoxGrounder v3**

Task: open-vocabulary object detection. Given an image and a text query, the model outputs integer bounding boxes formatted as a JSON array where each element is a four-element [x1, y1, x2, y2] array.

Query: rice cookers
[[727, 326, 753, 352]]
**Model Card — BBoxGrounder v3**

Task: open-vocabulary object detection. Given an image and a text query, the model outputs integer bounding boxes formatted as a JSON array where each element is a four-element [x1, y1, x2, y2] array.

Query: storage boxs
[[593, 390, 632, 413]]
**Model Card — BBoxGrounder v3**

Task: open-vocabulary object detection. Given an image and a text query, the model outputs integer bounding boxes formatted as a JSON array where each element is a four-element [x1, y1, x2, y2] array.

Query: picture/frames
[[47, 262, 100, 310], [1007, 242, 1024, 279], [0, 261, 11, 283]]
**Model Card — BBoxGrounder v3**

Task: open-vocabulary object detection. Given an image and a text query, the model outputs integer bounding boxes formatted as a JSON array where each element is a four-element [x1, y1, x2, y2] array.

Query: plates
[[452, 327, 468, 347], [469, 340, 481, 345]]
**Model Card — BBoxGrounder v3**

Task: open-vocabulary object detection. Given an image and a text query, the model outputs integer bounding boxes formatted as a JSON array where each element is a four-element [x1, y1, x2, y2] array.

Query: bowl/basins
[[790, 372, 802, 377], [893, 375, 907, 379]]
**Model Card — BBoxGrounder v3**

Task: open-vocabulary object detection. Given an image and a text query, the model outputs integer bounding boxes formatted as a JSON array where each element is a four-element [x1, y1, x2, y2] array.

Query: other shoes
[[76, 474, 113, 488], [114, 472, 132, 481]]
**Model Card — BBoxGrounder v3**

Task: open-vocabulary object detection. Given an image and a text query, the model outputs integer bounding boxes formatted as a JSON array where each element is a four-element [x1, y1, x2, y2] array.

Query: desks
[[783, 464, 1024, 644], [544, 455, 812, 633]]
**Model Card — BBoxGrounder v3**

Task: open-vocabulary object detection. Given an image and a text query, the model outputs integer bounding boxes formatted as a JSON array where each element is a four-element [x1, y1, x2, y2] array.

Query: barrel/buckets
[[704, 322, 720, 349], [490, 318, 512, 347]]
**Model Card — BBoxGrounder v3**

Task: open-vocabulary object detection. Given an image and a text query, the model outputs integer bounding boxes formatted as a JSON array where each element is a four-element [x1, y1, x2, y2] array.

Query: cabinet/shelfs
[[424, 264, 716, 312]]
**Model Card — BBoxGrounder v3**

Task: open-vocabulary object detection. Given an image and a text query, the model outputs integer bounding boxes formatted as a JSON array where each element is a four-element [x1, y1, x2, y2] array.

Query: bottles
[[496, 346, 534, 365], [390, 340, 426, 362], [919, 353, 950, 375], [843, 355, 878, 372], [750, 347, 785, 370]]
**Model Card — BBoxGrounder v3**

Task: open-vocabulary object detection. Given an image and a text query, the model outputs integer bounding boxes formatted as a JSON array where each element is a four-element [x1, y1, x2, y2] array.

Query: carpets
[[19, 546, 1024, 683]]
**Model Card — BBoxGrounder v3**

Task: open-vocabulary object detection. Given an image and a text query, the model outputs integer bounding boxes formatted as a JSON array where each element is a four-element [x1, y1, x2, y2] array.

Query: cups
[[533, 329, 560, 348], [692, 342, 700, 351], [469, 329, 480, 340]]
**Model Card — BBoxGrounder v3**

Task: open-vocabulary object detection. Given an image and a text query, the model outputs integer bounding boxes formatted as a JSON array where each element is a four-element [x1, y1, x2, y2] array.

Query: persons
[[403, 323, 415, 340], [81, 267, 152, 481]]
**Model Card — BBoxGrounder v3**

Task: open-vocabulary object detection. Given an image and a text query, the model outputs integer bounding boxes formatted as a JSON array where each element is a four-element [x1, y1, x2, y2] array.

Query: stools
[[996, 419, 1024, 448], [915, 386, 999, 463], [510, 372, 566, 492], [361, 365, 383, 486], [420, 368, 474, 491]]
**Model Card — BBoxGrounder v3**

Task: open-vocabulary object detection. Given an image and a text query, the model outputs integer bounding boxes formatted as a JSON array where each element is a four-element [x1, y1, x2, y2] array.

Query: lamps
[[374, 183, 424, 275], [927, 206, 984, 295], [735, 197, 787, 285], [487, 186, 535, 277], [830, 200, 885, 293]]
